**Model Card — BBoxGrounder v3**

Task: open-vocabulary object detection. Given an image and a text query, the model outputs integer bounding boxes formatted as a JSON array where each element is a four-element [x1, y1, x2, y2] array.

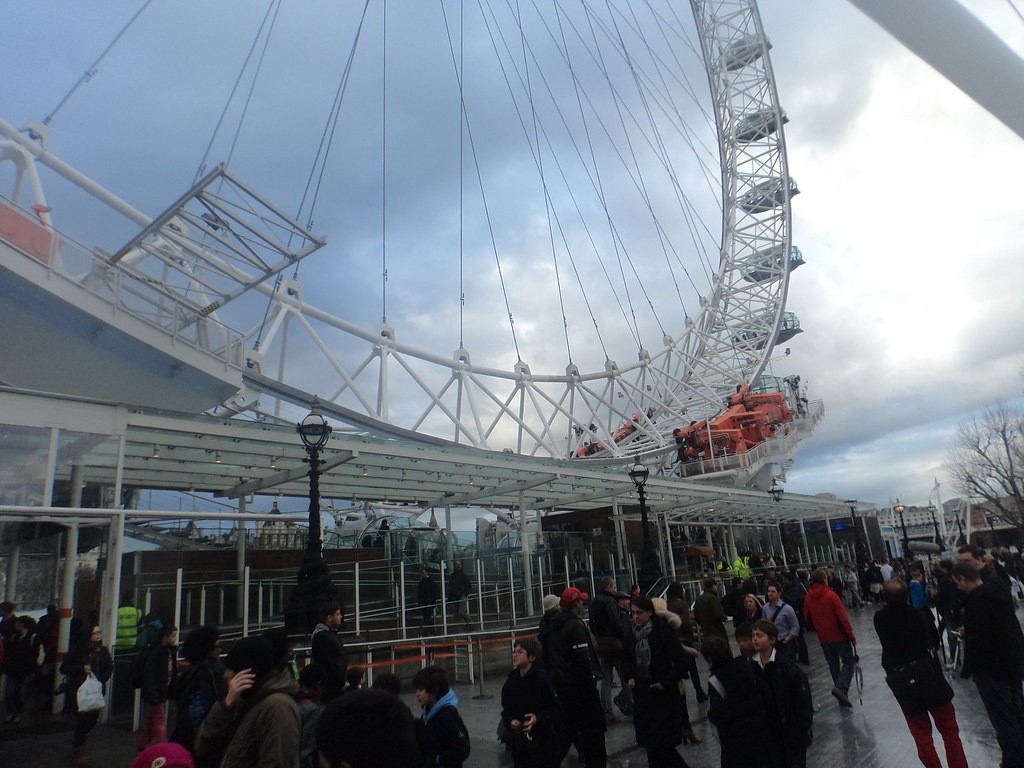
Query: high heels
[[682, 727, 702, 746]]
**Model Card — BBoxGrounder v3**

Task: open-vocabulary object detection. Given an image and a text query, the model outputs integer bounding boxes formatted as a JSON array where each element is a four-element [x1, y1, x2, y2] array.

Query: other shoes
[[946, 658, 956, 668], [4, 713, 20, 722], [613, 697, 630, 716], [832, 687, 852, 708], [73, 748, 87, 764], [604, 717, 620, 726]]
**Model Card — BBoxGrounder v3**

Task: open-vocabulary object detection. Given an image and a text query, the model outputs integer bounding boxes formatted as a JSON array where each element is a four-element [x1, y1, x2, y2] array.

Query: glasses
[[211, 642, 224, 651], [92, 631, 103, 635], [511, 650, 528, 656]]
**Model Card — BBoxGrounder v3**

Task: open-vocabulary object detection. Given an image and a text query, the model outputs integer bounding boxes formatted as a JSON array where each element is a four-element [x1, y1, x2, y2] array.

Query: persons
[[0, 598, 470, 768], [873, 577, 969, 768], [446, 560, 473, 634], [362, 520, 453, 570], [497, 639, 562, 768], [672, 384, 782, 462], [803, 570, 858, 709], [951, 561, 1024, 768], [536, 576, 812, 768], [59, 623, 114, 768], [569, 406, 660, 458], [417, 568, 441, 637], [540, 588, 607, 768], [718, 544, 1024, 615]]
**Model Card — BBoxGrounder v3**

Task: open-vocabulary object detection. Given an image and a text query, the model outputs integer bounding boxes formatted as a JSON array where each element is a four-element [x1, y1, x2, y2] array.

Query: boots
[[696, 687, 708, 703]]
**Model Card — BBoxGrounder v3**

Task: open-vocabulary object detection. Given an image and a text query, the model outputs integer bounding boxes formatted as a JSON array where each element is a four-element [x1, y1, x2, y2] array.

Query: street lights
[[844, 497, 870, 592], [983, 510, 1002, 549], [280, 388, 339, 637], [927, 500, 946, 554], [949, 504, 967, 546], [893, 497, 917, 560], [629, 451, 668, 599]]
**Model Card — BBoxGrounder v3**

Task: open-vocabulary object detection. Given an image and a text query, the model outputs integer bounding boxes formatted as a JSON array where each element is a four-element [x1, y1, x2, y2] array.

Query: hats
[[561, 587, 589, 605], [615, 591, 631, 599], [226, 628, 289, 680], [133, 742, 194, 768], [544, 594, 561, 611]]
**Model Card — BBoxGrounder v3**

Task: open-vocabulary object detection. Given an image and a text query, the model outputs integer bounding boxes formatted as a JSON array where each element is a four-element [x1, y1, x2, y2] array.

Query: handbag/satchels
[[76, 672, 105, 713], [885, 655, 954, 714], [597, 637, 623, 657]]
